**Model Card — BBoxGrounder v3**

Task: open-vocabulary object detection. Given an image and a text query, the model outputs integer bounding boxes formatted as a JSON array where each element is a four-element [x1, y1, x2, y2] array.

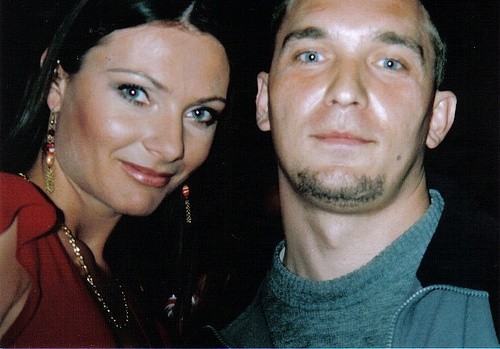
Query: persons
[[1, 0, 259, 349], [181, 0, 500, 348]]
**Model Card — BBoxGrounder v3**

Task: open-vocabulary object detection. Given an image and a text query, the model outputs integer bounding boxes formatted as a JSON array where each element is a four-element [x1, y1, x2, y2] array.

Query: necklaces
[[20, 171, 132, 331]]
